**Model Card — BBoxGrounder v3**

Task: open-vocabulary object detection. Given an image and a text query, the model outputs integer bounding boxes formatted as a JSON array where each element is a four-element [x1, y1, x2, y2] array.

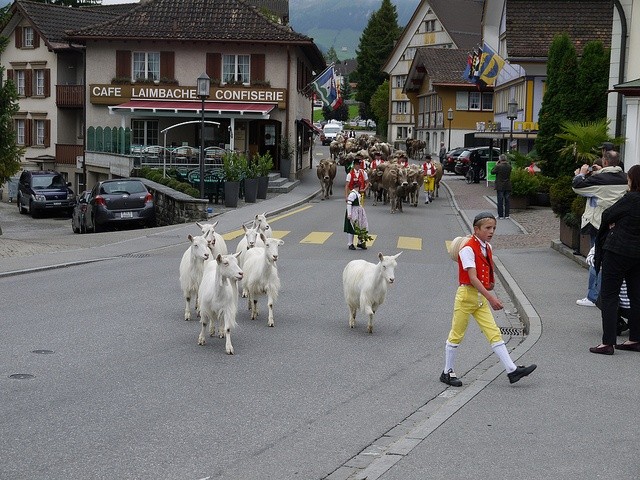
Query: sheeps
[[198, 251, 244, 355], [236, 223, 265, 298], [343, 251, 405, 333], [251, 211, 272, 238], [242, 233, 284, 326], [196, 219, 228, 260], [179, 230, 214, 321]]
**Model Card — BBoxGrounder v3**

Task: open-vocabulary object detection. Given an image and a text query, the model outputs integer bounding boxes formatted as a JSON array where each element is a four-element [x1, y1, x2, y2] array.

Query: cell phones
[[587, 167, 597, 172]]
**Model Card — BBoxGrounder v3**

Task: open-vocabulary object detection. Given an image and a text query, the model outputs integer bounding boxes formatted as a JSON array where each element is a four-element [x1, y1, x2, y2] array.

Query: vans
[[323, 123, 340, 146]]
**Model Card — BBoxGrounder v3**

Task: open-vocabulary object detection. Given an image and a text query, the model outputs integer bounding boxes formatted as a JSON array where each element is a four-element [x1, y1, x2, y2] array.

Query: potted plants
[[278, 129, 295, 178], [223, 149, 243, 208], [243, 154, 260, 202], [258, 150, 273, 199]]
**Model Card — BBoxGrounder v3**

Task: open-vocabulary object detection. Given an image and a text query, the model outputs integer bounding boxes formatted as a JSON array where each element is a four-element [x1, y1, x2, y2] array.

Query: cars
[[71, 191, 90, 233], [85, 178, 155, 229]]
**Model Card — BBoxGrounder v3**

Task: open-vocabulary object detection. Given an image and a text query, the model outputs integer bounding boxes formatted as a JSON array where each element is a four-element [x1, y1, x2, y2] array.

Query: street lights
[[448, 108, 453, 152], [507, 97, 518, 151], [196, 70, 211, 198]]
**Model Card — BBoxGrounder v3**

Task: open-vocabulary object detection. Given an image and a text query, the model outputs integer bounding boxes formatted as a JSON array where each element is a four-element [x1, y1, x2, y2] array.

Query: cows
[[330, 133, 443, 214], [316, 159, 337, 200]]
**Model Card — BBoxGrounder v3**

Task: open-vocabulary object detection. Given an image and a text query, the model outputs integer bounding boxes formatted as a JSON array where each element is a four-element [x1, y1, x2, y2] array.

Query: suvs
[[454, 147, 500, 179], [17, 169, 76, 217], [442, 148, 467, 175]]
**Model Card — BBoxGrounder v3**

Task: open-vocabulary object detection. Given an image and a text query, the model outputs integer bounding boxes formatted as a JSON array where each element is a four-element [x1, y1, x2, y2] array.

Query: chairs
[[176, 166, 228, 203]]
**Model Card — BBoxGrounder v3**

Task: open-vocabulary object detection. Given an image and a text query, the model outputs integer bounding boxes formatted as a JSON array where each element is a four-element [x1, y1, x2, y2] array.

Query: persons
[[575, 230, 610, 308], [470, 151, 483, 184], [421, 156, 437, 204], [491, 154, 513, 220], [351, 154, 370, 170], [370, 153, 385, 169], [589, 165, 640, 355], [440, 211, 538, 387], [439, 142, 446, 174], [348, 128, 356, 138], [572, 150, 631, 335], [344, 159, 369, 208], [344, 178, 369, 251]]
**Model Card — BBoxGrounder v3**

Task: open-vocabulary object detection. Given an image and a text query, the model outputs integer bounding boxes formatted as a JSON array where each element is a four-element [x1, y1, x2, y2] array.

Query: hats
[[354, 159, 361, 163], [598, 142, 614, 150], [473, 212, 495, 227], [425, 156, 432, 159]]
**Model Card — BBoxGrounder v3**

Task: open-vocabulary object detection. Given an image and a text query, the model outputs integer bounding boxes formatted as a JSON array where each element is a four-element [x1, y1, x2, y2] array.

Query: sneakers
[[505, 217, 509, 219], [617, 318, 628, 335], [495, 216, 503, 219], [577, 297, 596, 306]]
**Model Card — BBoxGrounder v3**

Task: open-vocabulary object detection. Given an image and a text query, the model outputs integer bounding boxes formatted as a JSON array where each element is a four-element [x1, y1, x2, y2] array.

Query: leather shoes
[[590, 344, 614, 355], [349, 244, 356, 250], [429, 197, 432, 202], [441, 370, 462, 387], [426, 201, 428, 204], [357, 243, 367, 249], [615, 341, 640, 352], [508, 364, 537, 384]]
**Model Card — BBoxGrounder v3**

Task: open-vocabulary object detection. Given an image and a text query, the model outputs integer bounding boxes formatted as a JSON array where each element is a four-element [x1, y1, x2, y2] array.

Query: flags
[[309, 65, 337, 107], [313, 72, 343, 112], [479, 42, 505, 86], [463, 47, 488, 88]]
